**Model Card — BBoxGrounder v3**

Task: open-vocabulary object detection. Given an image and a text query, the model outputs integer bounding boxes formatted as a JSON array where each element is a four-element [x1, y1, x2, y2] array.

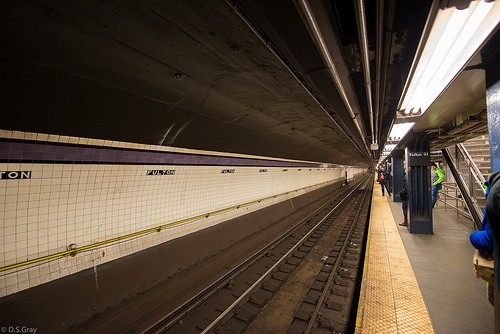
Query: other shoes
[[399, 221, 407, 225]]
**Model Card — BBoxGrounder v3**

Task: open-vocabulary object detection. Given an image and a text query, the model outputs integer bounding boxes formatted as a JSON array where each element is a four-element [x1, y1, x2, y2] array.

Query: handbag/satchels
[[380, 173, 384, 182], [472, 249, 495, 287]]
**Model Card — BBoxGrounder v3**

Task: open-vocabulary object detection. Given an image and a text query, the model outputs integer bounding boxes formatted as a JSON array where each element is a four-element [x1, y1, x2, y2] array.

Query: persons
[[469, 171, 500, 334], [431, 164, 445, 208], [398, 169, 408, 226], [376, 169, 392, 196]]
